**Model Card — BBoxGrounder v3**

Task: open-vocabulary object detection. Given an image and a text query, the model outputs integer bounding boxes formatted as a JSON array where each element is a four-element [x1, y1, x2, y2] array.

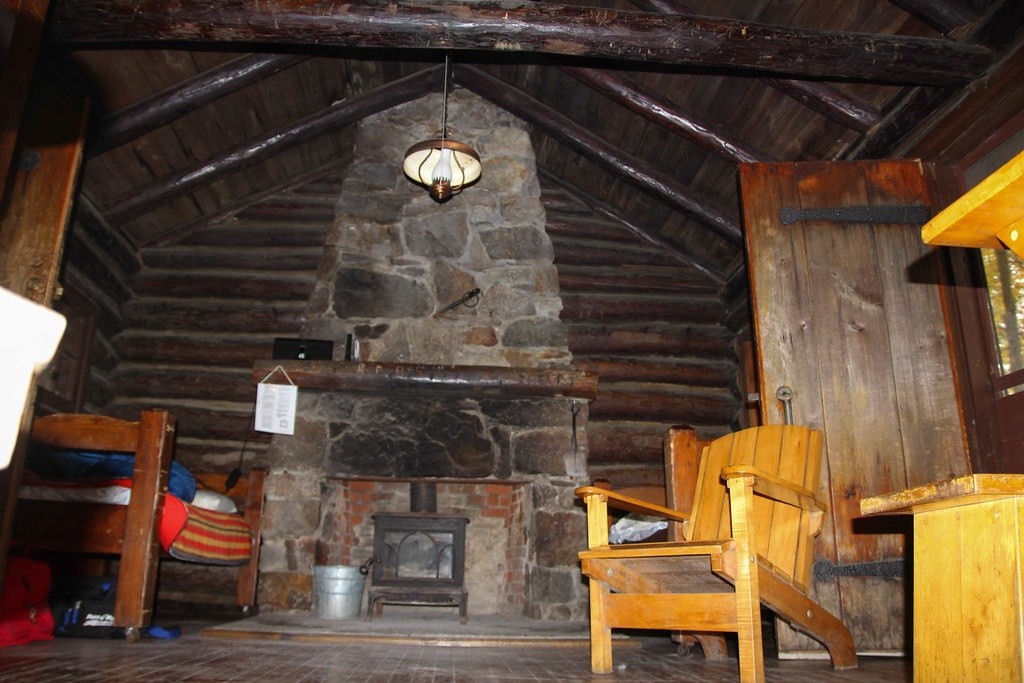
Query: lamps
[[403, 55, 482, 200]]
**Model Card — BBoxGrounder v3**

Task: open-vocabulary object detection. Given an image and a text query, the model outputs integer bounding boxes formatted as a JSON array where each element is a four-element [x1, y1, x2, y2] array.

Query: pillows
[[188, 491, 238, 515]]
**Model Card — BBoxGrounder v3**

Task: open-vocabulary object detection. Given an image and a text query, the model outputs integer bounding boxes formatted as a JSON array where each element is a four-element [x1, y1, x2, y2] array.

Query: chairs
[[574, 424, 858, 683]]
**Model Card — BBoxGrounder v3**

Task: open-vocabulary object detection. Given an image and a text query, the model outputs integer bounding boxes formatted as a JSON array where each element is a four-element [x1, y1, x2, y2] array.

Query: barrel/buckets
[[309, 563, 367, 620]]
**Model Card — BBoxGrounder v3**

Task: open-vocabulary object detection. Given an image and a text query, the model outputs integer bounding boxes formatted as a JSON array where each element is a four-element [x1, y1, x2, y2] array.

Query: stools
[[859, 473, 1023, 683]]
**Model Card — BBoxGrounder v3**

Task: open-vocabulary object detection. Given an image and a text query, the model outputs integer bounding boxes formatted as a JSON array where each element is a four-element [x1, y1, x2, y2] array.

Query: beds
[[5, 409, 268, 627]]
